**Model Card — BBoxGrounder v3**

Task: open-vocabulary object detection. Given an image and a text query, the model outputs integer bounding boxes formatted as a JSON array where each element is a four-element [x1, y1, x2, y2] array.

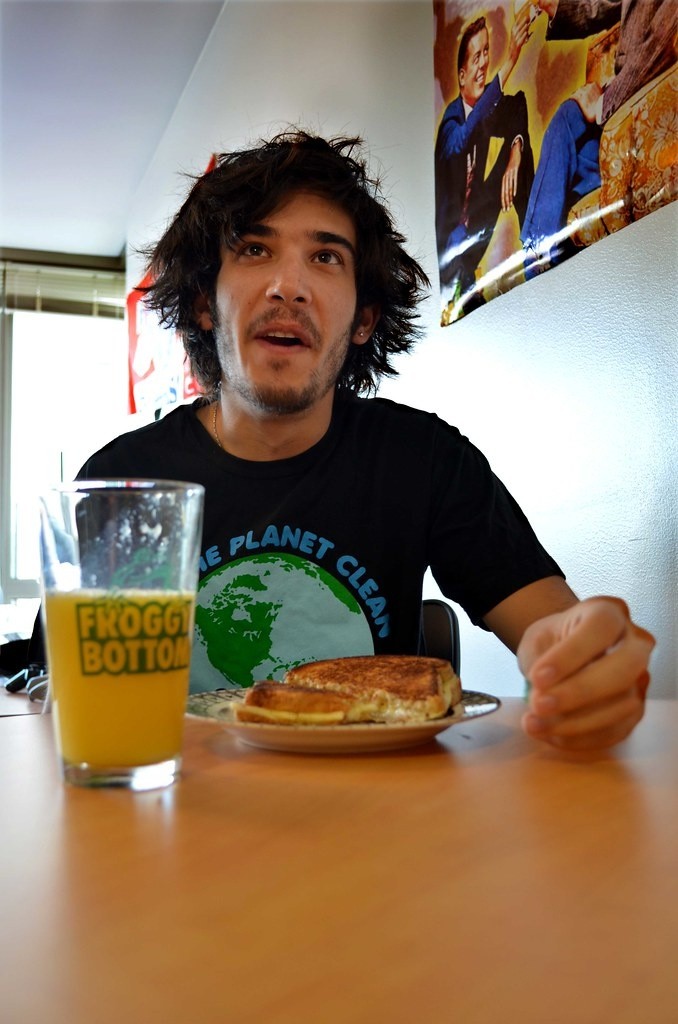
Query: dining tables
[[1, 697, 678, 1024]]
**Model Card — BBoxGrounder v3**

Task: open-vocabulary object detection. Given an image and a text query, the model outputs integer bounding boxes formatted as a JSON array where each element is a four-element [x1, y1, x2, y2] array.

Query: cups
[[37, 479, 203, 792]]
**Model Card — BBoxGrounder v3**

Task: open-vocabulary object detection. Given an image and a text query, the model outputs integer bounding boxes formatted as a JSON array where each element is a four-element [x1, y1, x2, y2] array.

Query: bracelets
[[512, 137, 522, 148]]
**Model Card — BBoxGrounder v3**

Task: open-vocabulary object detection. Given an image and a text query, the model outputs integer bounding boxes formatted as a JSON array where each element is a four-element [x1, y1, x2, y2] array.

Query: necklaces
[[212, 399, 221, 445]]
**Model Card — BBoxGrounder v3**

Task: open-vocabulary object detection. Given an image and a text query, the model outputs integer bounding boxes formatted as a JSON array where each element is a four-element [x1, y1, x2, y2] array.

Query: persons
[[520, 0, 678, 281], [433, 0, 535, 316], [27, 132, 654, 751]]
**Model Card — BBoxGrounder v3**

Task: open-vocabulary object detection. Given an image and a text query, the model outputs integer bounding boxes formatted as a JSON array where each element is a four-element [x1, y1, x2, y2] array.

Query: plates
[[183, 685, 500, 751]]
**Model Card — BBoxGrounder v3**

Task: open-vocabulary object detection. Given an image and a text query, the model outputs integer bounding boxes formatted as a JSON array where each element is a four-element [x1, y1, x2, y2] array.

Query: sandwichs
[[283, 654, 464, 721], [228, 680, 412, 726]]
